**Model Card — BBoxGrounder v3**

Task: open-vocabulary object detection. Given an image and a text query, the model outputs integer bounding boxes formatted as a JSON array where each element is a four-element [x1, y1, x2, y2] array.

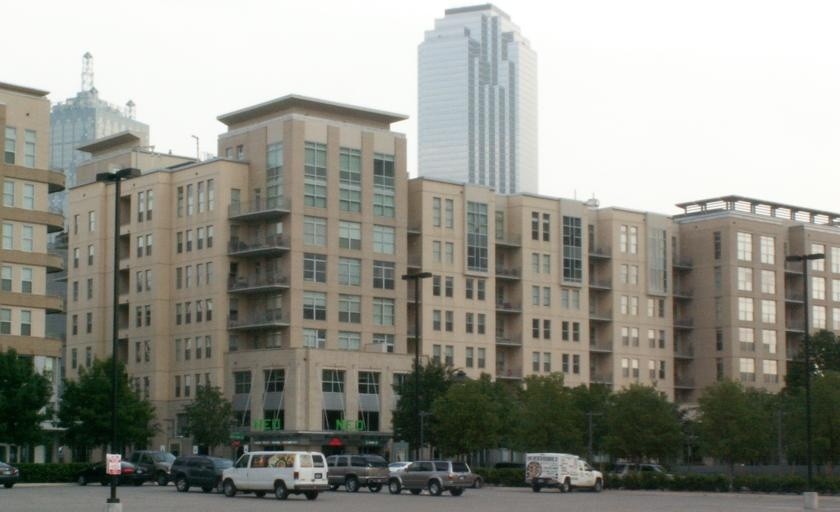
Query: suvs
[[170, 454, 232, 492], [388, 460, 472, 495], [128, 451, 176, 487]]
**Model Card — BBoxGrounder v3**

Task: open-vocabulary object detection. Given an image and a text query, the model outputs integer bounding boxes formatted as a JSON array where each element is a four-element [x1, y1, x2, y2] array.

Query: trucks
[[524, 452, 605, 492]]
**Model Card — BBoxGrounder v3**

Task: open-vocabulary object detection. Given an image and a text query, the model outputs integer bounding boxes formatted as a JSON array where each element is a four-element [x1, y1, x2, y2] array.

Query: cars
[[78, 460, 148, 486], [469, 473, 484, 489], [608, 462, 675, 481], [388, 460, 413, 470], [0, 461, 21, 489]]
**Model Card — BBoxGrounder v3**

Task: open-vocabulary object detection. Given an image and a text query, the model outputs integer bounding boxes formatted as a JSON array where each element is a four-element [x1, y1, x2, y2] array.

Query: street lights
[[786, 252, 826, 510], [94, 165, 142, 504]]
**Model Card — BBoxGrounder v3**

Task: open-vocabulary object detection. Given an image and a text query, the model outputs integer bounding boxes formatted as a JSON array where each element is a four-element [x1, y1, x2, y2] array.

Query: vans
[[325, 453, 388, 492], [221, 450, 329, 501], [402, 271, 433, 460]]
